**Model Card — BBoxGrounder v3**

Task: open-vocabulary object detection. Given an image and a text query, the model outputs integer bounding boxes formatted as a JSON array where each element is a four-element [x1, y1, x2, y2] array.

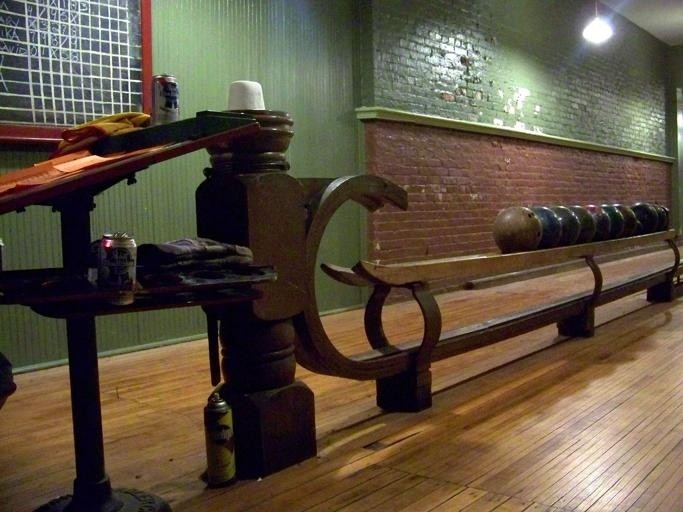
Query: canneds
[[151, 75, 179, 126], [98, 233, 137, 305]]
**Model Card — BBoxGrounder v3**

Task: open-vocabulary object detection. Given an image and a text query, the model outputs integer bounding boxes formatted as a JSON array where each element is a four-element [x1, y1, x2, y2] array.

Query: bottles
[[202, 390, 237, 488]]
